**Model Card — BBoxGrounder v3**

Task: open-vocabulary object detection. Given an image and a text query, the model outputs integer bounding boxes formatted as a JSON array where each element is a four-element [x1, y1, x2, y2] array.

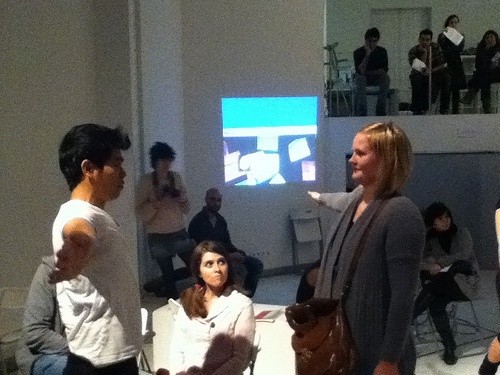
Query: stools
[[327, 89, 355, 116], [365, 86, 389, 115]]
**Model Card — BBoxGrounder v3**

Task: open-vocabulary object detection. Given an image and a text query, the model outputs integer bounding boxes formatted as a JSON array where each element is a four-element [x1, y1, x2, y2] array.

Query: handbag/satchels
[[285, 297, 356, 375]]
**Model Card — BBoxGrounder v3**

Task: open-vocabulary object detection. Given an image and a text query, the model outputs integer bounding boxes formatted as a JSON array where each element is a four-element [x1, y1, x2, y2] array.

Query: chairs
[[416, 296, 481, 352], [291, 210, 324, 275]]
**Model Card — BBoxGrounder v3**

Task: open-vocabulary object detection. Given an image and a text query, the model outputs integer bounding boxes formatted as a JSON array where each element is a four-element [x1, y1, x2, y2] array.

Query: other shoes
[[444, 345, 456, 364], [461, 93, 472, 104], [143, 281, 168, 297]]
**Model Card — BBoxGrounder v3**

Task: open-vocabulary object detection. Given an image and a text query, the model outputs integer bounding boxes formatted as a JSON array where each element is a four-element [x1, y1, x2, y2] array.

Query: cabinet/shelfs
[[458, 55, 480, 114]]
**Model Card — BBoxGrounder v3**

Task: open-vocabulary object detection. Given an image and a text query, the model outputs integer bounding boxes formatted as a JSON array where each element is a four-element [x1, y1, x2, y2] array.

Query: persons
[[15, 254, 69, 375], [170, 240, 255, 375], [138, 142, 197, 299], [308, 123, 425, 375], [353, 27, 390, 115], [478, 207, 500, 375], [409, 28, 441, 116], [460, 30, 500, 114], [48, 123, 142, 375], [188, 188, 263, 299], [437, 15, 466, 115], [411, 202, 480, 365]]
